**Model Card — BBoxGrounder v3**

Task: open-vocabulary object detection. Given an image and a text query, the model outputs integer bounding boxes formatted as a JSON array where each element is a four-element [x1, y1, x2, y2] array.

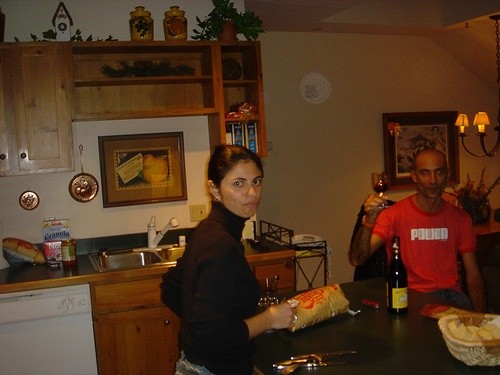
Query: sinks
[[88, 249, 163, 273], [155, 247, 185, 262]]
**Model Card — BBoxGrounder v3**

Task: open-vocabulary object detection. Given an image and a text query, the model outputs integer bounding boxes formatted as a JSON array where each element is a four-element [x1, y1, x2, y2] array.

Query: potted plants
[[190, 0, 266, 42]]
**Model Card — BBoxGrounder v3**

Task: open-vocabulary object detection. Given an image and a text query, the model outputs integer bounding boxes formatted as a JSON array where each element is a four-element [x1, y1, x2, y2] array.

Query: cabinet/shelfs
[[92, 259, 296, 375], [208, 39, 267, 158], [0, 41, 78, 176], [73, 37, 218, 121]]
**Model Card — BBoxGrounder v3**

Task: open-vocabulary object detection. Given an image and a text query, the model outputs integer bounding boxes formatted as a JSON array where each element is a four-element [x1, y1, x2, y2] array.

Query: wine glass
[[371, 170, 389, 208]]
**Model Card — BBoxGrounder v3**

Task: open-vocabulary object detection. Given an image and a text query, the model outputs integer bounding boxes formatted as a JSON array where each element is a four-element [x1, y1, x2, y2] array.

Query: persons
[[349, 148, 487, 313], [160, 145, 297, 375]]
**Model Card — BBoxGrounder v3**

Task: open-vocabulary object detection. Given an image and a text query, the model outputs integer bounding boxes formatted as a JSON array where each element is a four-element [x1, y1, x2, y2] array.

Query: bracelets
[[363, 216, 373, 228]]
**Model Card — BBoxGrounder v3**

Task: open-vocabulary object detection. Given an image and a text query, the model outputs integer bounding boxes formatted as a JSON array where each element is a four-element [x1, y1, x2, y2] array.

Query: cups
[[257, 275, 279, 307]]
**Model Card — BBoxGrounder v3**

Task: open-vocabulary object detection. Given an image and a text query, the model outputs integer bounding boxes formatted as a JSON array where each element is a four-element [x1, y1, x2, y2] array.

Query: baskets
[[438, 312, 500, 366]]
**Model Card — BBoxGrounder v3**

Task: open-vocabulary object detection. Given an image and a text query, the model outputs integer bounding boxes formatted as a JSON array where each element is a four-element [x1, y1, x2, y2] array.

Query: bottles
[[60, 238, 77, 268], [385, 236, 409, 314], [128, 6, 153, 42], [162, 5, 187, 41]]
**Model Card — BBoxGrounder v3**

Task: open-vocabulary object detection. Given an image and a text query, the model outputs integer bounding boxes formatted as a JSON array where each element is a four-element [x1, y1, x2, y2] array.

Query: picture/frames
[[382, 110, 461, 189], [98, 130, 188, 208]]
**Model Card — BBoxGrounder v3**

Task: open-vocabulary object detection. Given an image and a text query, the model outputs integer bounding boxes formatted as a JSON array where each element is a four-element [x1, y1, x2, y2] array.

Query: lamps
[[455, 14, 500, 158]]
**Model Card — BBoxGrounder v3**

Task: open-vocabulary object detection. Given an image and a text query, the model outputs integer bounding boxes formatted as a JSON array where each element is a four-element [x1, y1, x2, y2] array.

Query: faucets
[[147, 215, 180, 249]]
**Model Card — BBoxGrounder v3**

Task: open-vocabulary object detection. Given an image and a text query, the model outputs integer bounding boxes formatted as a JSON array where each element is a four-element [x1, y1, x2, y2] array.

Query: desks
[[459, 209, 500, 264], [245, 277, 500, 375]]
